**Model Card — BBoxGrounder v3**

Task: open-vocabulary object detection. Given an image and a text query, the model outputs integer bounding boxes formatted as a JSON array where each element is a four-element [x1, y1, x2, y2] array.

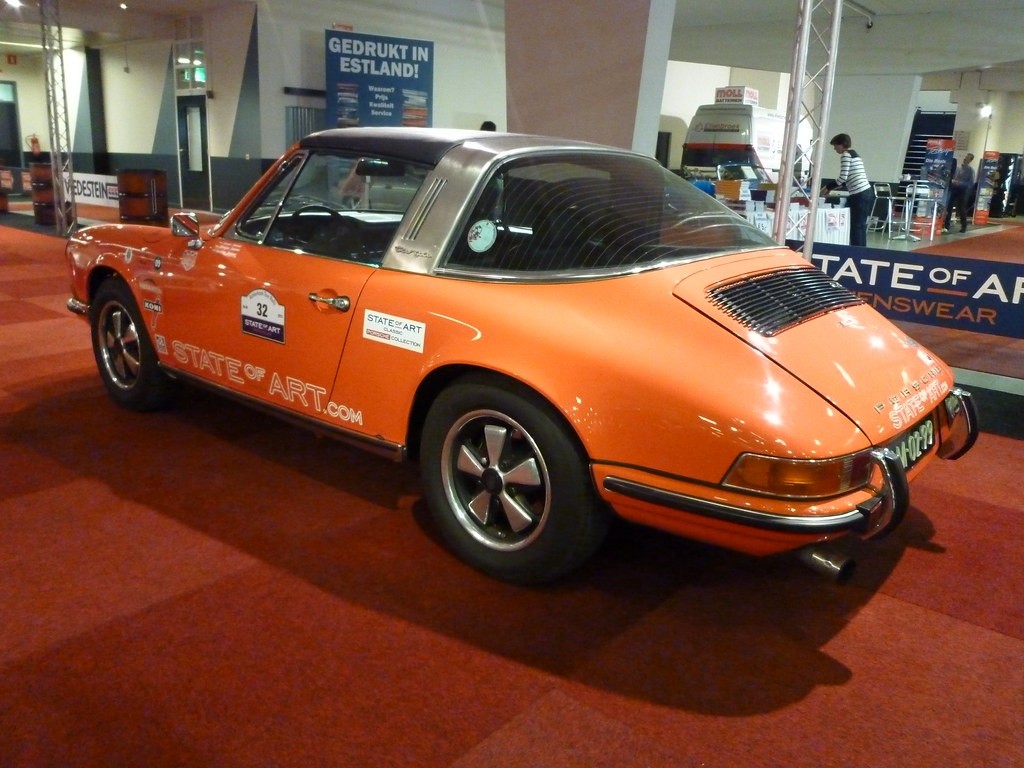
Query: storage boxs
[[716, 85, 758, 105], [715, 181, 846, 211]]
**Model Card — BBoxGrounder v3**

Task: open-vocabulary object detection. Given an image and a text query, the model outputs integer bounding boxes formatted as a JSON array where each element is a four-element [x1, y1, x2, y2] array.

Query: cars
[[67, 127, 980, 585]]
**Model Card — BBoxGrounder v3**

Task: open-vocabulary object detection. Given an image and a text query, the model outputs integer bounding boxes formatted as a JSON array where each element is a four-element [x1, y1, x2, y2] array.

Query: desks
[[733, 207, 850, 244], [892, 180, 930, 242]]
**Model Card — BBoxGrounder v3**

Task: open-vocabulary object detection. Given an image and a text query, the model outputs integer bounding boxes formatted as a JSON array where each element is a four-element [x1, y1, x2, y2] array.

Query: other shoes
[[959, 228, 966, 234], [941, 228, 948, 235]]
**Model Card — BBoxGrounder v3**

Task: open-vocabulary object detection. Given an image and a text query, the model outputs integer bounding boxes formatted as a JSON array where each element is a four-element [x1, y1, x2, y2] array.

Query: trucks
[[681, 86, 813, 187]]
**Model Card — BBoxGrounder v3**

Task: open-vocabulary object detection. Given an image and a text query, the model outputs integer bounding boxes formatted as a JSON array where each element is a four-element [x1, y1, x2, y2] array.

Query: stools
[[905, 185, 937, 242], [867, 183, 908, 240]]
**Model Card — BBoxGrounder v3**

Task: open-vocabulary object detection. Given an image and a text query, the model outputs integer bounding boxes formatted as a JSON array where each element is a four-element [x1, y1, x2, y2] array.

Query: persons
[[938, 153, 975, 232], [481, 120, 504, 216], [821, 133, 876, 246]]
